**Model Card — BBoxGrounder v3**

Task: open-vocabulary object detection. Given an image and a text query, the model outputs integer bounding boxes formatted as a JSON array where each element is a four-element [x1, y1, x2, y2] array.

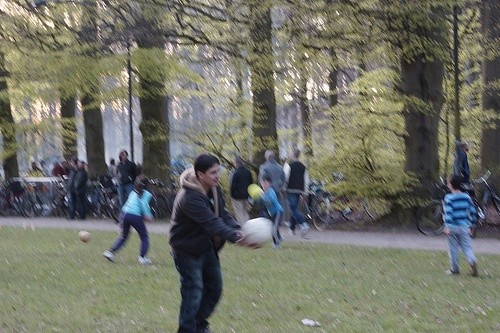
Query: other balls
[[80, 230, 91, 243], [247, 183, 261, 197], [244, 216, 273, 244]]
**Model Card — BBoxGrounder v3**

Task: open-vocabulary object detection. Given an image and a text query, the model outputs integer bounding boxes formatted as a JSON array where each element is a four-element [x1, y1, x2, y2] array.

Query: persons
[[443, 176, 478, 277], [283, 149, 309, 235], [260, 176, 284, 246], [102, 177, 154, 267], [169, 154, 262, 333], [65, 156, 86, 221], [114, 150, 142, 206], [452, 137, 470, 193], [108, 158, 118, 177], [32, 156, 75, 176], [229, 158, 252, 229], [259, 150, 285, 201]]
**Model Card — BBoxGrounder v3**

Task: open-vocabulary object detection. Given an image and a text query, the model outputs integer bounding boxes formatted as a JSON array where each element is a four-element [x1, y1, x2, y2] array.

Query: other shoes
[[103, 250, 116, 263], [138, 256, 151, 264]]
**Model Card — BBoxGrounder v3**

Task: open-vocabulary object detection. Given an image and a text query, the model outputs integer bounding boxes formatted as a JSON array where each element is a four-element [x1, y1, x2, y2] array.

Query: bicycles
[[471, 171, 500, 227], [0, 172, 193, 223], [298, 172, 380, 232], [415, 174, 479, 238]]
